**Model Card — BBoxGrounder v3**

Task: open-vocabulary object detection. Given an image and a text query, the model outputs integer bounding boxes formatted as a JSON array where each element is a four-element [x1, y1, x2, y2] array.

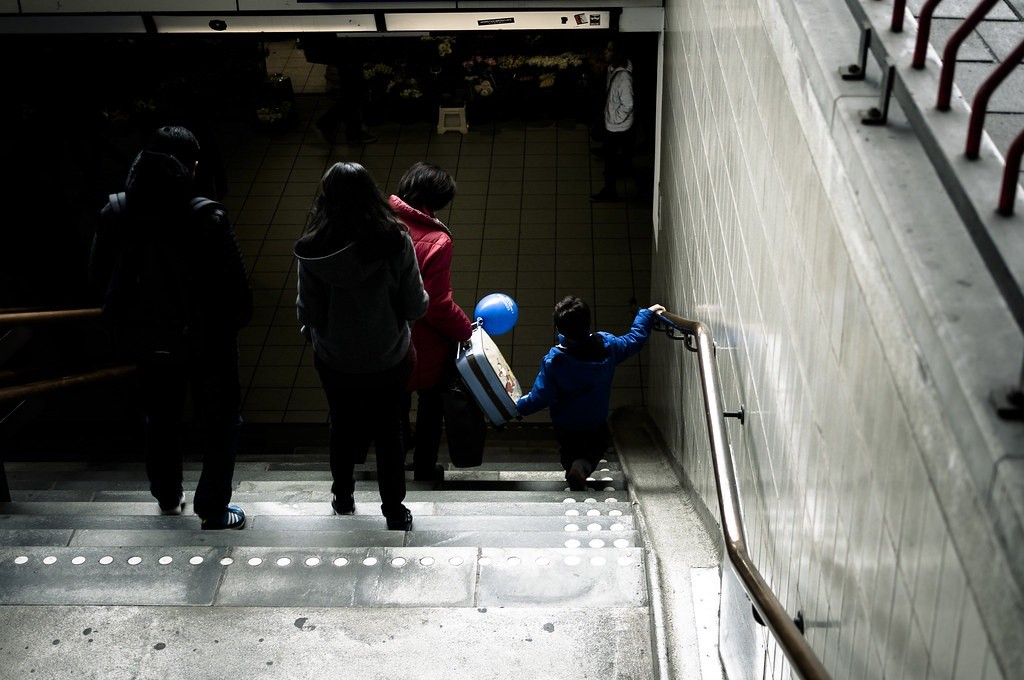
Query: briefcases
[[456, 316, 524, 427]]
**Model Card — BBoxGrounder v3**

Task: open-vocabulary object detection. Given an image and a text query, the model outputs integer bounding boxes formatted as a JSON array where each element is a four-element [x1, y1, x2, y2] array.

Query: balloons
[[474, 293, 518, 336]]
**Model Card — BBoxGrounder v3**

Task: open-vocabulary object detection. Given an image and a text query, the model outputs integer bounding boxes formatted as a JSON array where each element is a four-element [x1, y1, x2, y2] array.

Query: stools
[[437, 102, 469, 134]]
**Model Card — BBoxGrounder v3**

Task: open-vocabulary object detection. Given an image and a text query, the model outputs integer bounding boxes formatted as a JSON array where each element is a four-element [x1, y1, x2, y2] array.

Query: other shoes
[[590, 191, 614, 203], [401, 510, 413, 532], [591, 146, 606, 159], [569, 461, 586, 492], [331, 494, 355, 516], [349, 132, 377, 143], [316, 120, 336, 144], [426, 463, 445, 483]]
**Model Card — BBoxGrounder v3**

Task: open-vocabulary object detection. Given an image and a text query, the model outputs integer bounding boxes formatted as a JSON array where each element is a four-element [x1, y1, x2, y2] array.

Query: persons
[[589, 39, 634, 203], [518, 296, 666, 491], [313, 64, 377, 143], [89, 126, 253, 531], [294, 163, 429, 531], [388, 161, 472, 482]]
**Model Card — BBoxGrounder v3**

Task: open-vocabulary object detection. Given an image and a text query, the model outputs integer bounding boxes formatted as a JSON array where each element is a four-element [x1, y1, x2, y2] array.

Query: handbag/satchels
[[444, 375, 487, 467]]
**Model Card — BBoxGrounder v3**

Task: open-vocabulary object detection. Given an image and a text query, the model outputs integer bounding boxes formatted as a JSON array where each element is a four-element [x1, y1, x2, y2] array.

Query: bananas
[[528, 52, 582, 87]]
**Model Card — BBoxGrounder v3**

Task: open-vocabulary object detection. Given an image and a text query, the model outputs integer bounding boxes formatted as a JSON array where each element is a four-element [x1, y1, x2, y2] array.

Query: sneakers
[[161, 492, 186, 516], [200, 505, 246, 531]]
